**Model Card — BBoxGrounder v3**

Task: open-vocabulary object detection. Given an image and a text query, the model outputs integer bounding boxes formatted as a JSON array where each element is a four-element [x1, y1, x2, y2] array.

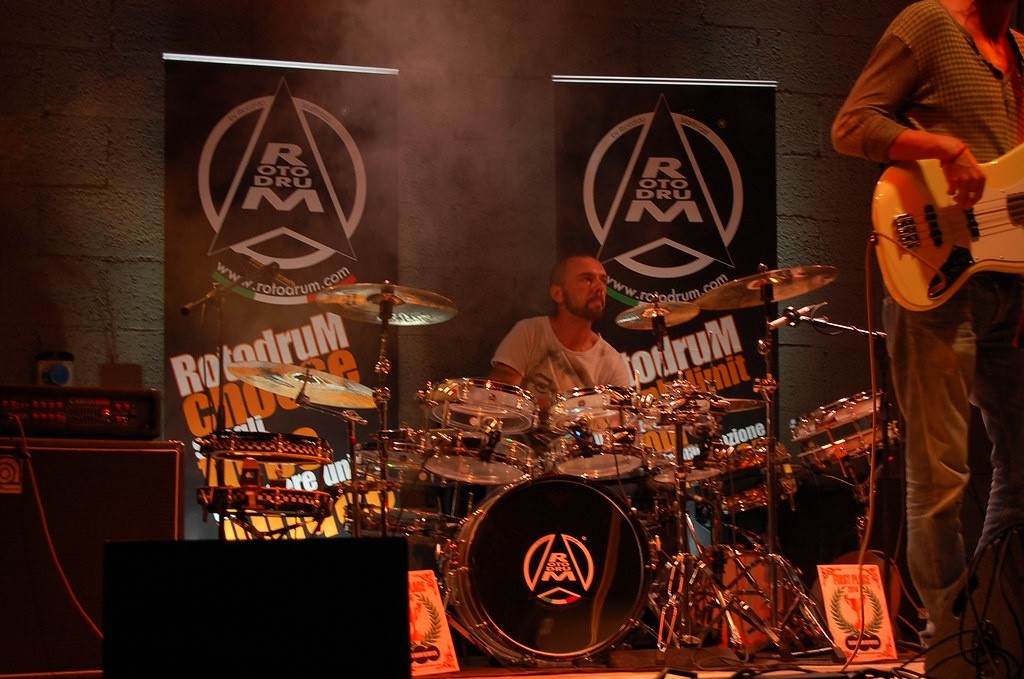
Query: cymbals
[[615, 293, 701, 331], [703, 379, 764, 415], [653, 466, 723, 484], [693, 262, 840, 311], [224, 359, 377, 411], [314, 279, 459, 328]]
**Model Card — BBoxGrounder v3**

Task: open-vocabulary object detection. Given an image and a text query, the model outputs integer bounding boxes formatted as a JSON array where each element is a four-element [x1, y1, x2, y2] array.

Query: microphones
[[768, 303, 817, 330]]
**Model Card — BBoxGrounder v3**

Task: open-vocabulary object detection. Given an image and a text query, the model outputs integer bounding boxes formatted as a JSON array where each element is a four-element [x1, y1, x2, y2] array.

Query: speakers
[[103, 535, 412, 678], [0, 435, 186, 678]]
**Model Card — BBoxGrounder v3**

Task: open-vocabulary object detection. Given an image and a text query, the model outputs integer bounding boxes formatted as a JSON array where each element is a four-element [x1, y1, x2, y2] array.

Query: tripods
[[647, 295, 932, 679]]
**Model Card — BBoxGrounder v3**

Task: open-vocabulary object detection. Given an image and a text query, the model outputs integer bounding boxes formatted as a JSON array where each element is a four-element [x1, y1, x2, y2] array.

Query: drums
[[648, 548, 790, 657], [427, 375, 541, 437], [194, 485, 332, 519], [788, 387, 898, 472], [361, 427, 431, 472], [709, 444, 798, 514], [438, 469, 655, 666], [196, 429, 334, 467], [420, 434, 536, 487], [349, 504, 464, 602], [548, 383, 640, 435], [550, 432, 646, 481]]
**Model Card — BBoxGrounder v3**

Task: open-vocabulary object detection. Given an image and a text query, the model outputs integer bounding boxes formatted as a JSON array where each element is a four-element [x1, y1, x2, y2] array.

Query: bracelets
[[939, 143, 969, 168]]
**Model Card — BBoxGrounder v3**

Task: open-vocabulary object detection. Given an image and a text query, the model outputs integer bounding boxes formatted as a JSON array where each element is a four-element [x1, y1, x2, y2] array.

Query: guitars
[[869, 140, 1024, 312]]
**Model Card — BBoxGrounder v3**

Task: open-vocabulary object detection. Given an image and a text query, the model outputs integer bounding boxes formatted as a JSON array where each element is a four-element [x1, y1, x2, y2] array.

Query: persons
[[488, 256, 634, 411], [831, 0, 1024, 679]]
